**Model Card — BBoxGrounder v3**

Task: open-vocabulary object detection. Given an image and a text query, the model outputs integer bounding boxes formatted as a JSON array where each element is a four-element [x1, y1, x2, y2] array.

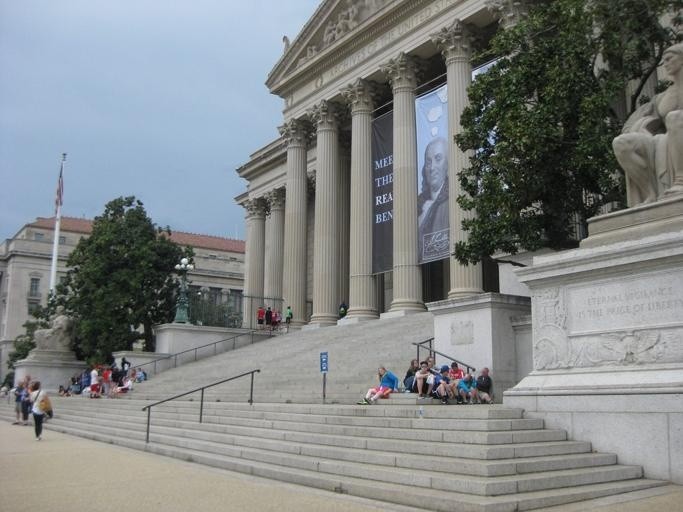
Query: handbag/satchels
[[38, 397, 53, 418]]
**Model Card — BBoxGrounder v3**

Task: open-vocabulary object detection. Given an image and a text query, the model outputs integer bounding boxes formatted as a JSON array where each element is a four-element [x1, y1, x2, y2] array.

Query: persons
[[265, 307, 272, 329], [339, 301, 348, 317], [416, 135, 449, 261], [276, 311, 282, 330], [256, 306, 265, 330], [610, 41, 683, 207], [28, 379, 49, 441], [355, 366, 398, 404], [318, 4, 359, 47], [403, 355, 494, 405], [285, 306, 293, 329], [57, 355, 148, 399], [12, 374, 31, 424], [271, 308, 278, 330]]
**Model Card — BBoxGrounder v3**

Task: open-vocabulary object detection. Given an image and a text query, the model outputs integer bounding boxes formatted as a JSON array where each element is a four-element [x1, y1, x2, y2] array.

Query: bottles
[[417, 404, 425, 420]]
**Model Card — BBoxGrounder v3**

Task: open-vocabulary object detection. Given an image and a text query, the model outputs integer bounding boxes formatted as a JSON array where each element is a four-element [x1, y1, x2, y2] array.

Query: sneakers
[[36, 434, 42, 441], [357, 398, 374, 404], [418, 394, 434, 400], [442, 398, 493, 404], [12, 421, 29, 425]]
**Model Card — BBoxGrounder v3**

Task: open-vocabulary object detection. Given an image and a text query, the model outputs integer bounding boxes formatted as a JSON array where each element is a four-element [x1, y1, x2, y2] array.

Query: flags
[[53, 156, 65, 218]]
